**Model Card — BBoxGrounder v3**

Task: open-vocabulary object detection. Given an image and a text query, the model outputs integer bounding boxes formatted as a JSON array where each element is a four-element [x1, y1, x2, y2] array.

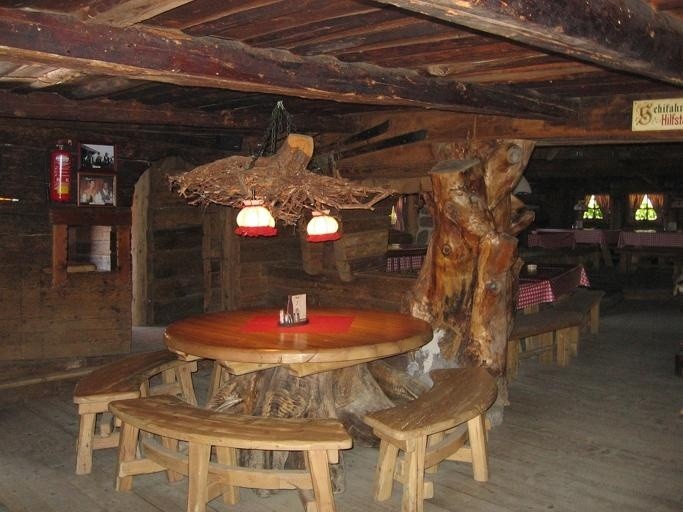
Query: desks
[[524, 226, 681, 245], [388, 245, 427, 272], [516, 261, 582, 316], [162, 303, 432, 496]]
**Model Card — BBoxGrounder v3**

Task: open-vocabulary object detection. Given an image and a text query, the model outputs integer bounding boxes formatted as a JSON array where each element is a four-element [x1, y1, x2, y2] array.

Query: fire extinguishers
[[50, 143, 73, 203]]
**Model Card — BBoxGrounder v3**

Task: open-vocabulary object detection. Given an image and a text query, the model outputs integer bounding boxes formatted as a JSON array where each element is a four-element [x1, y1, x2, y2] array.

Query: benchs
[[522, 247, 682, 296], [361, 365, 501, 511], [106, 394, 355, 512], [73, 349, 199, 492], [505, 289, 609, 384]]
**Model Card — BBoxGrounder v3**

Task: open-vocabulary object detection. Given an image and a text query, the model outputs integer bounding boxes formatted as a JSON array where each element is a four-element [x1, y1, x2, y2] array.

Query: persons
[[82, 179, 97, 202], [97, 181, 111, 201]]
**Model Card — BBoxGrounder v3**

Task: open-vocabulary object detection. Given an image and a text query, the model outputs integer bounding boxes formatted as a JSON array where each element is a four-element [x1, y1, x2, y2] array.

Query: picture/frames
[[76, 141, 119, 209]]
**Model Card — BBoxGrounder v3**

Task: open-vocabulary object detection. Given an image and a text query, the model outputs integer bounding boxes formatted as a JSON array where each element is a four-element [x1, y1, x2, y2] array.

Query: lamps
[[165, 100, 389, 247]]
